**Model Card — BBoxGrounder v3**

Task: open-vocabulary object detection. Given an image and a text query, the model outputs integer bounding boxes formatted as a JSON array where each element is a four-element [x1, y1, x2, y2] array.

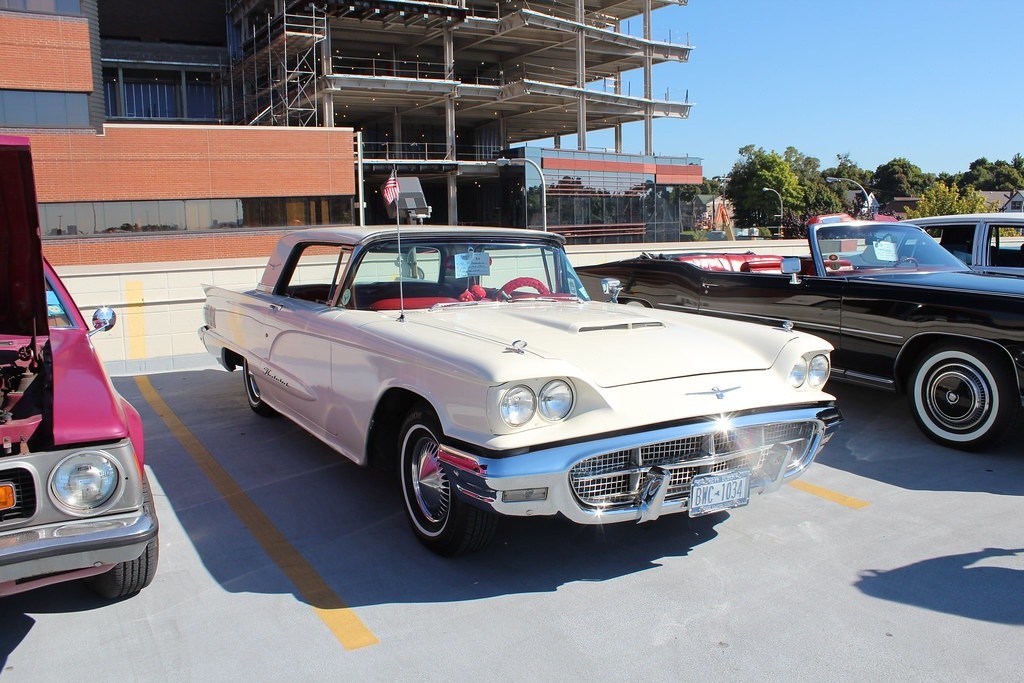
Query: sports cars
[[196, 224, 846, 558], [572, 214, 1023, 454]]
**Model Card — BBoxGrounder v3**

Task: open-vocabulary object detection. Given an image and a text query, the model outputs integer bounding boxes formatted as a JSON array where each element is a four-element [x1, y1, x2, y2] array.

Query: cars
[[823, 211, 1023, 277], [0, 131, 162, 603]]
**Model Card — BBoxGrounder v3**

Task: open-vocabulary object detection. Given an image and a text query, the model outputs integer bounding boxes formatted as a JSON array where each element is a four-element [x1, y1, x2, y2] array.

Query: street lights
[[762, 187, 783, 236], [825, 176, 871, 220], [496, 156, 548, 231], [718, 176, 732, 231]]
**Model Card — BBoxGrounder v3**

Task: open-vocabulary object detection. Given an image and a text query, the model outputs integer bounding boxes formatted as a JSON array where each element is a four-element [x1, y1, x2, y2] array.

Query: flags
[[383, 163, 401, 205]]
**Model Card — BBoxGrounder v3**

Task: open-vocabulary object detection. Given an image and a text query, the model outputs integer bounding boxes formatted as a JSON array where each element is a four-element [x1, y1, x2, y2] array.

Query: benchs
[[299, 283, 455, 309], [681, 254, 783, 272]]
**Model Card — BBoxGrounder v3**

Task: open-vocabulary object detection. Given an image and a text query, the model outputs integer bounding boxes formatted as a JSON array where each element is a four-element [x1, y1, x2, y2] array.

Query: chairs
[[823, 259, 853, 271], [739, 260, 782, 272]]
